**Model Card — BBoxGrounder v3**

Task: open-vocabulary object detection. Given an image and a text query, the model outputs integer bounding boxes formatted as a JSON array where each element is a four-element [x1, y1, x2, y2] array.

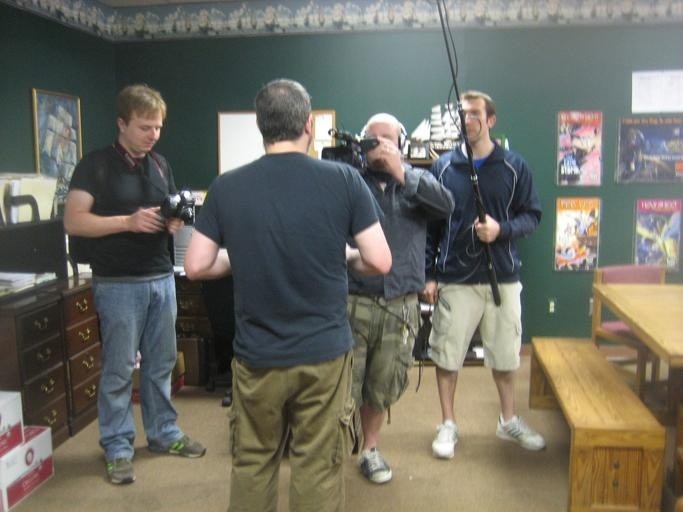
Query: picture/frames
[[614, 115, 683, 186], [218, 110, 335, 173], [32, 88, 83, 175]]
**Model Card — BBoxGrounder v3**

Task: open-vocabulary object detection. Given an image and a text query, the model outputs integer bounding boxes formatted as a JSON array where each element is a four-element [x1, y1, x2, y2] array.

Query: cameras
[[161, 191, 196, 225], [321, 129, 380, 175]]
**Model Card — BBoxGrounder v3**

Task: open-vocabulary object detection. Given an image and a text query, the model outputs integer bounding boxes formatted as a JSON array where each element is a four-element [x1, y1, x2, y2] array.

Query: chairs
[[594, 266, 665, 396]]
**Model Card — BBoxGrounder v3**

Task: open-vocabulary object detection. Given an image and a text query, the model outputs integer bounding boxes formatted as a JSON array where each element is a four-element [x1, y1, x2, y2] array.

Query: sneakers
[[359, 448, 392, 484], [432, 419, 458, 459], [106, 458, 136, 484], [148, 435, 206, 458], [495, 412, 546, 451]]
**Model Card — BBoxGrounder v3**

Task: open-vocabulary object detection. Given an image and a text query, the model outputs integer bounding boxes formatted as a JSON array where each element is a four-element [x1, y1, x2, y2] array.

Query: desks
[[592, 284, 683, 369]]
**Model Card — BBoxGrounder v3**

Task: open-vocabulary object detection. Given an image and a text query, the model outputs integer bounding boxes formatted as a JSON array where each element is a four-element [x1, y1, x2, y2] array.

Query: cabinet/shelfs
[[1, 277, 103, 448], [172, 275, 211, 341]]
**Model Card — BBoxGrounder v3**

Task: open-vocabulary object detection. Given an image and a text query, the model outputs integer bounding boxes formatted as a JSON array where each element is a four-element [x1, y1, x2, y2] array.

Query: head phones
[[360, 113, 408, 151]]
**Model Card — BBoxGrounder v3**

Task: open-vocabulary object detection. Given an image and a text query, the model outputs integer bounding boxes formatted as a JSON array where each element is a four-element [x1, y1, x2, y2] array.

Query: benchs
[[528, 336, 667, 512]]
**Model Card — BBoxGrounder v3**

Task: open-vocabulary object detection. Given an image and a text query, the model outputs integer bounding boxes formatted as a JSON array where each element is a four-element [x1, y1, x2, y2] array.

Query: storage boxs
[[0, 390, 25, 456], [132, 352, 186, 406], [0, 427, 55, 510]]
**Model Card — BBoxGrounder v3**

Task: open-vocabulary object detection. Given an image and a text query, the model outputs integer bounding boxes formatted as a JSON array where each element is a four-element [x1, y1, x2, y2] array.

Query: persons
[[422, 92, 547, 459], [63, 85, 207, 484], [569, 120, 590, 161], [347, 114, 455, 484], [184, 80, 392, 512]]
